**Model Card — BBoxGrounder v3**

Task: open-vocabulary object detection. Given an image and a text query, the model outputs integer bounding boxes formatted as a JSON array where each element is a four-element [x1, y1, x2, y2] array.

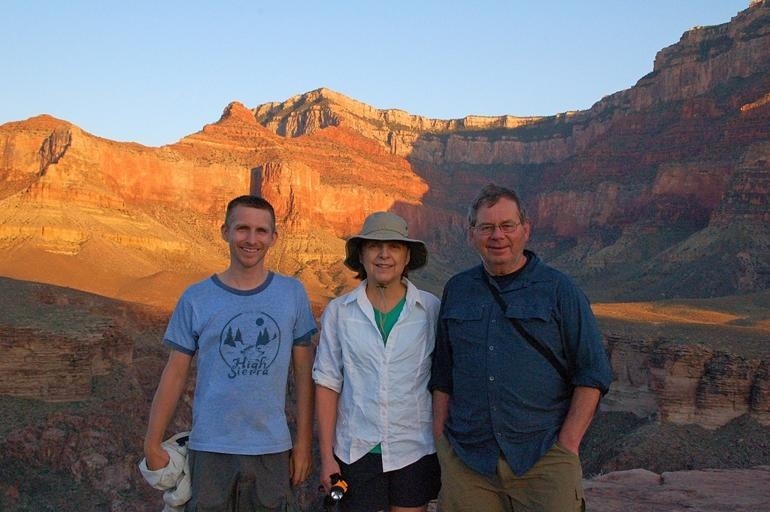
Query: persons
[[311, 211, 441, 512], [427, 181, 613, 512], [143, 195, 319, 512]]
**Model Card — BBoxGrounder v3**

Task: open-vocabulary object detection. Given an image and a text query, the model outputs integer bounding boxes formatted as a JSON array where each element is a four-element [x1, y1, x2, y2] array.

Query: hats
[[343, 211, 428, 275]]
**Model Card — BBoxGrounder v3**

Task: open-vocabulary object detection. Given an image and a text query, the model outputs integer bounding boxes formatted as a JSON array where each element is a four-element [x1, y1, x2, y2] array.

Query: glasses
[[470, 218, 523, 235]]
